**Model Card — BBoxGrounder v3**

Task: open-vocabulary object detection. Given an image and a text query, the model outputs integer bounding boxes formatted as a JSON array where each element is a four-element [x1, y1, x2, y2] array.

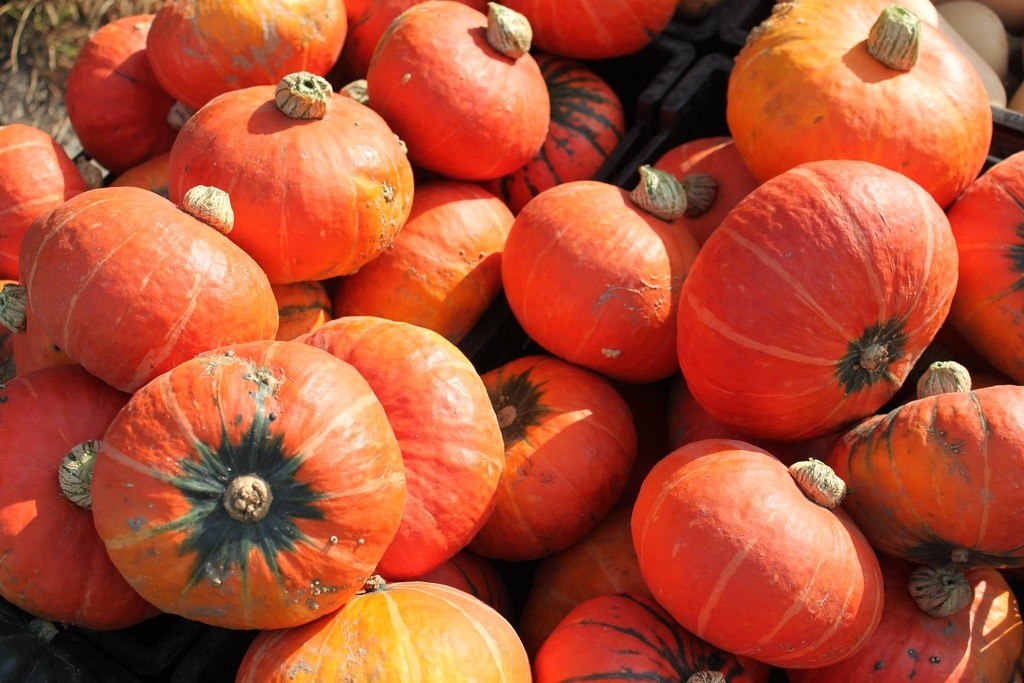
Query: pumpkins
[[0, 0, 1024, 683]]
